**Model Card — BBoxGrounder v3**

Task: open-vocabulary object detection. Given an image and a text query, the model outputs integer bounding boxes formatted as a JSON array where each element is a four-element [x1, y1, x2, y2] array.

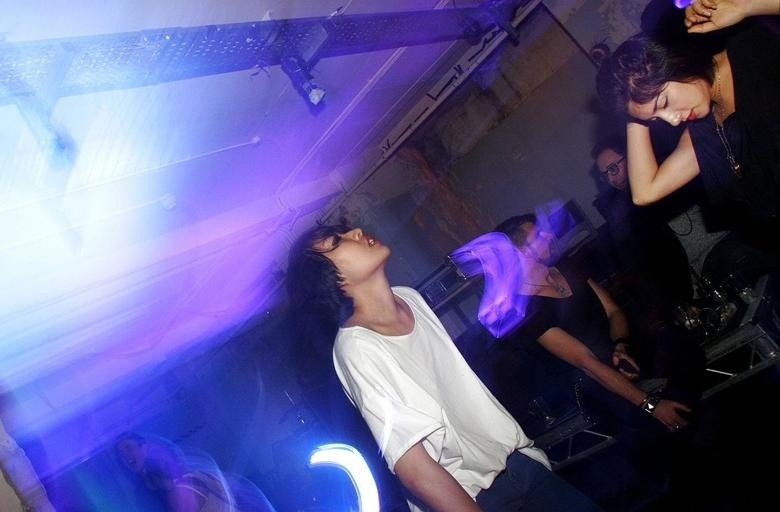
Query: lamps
[[282, 47, 327, 108]]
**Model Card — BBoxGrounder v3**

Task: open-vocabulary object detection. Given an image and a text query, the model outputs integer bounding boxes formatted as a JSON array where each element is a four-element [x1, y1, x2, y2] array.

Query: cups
[[531, 396, 556, 425], [723, 271, 756, 304]]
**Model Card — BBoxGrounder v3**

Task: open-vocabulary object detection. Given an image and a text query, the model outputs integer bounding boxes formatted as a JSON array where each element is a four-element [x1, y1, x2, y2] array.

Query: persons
[[593, 2, 779, 259], [143, 436, 237, 510], [589, 126, 762, 337], [114, 428, 277, 511], [284, 223, 556, 512], [495, 208, 693, 434]]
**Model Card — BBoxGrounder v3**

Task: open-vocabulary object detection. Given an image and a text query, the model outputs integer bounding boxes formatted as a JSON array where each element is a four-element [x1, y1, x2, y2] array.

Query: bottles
[[705, 280, 727, 305], [678, 305, 701, 329]]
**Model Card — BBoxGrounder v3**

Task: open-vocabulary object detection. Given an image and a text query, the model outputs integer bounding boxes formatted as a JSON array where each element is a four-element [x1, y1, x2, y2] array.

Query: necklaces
[[514, 269, 568, 294], [709, 65, 745, 180]]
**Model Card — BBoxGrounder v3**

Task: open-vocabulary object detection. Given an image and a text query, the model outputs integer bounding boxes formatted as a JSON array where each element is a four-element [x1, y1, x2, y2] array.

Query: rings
[[674, 423, 682, 431]]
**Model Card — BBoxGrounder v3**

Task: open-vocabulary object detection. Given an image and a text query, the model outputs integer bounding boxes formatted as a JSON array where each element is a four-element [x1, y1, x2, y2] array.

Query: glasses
[[598, 155, 627, 184]]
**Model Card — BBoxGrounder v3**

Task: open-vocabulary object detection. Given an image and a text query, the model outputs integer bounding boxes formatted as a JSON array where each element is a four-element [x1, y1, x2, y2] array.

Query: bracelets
[[636, 392, 661, 416]]
